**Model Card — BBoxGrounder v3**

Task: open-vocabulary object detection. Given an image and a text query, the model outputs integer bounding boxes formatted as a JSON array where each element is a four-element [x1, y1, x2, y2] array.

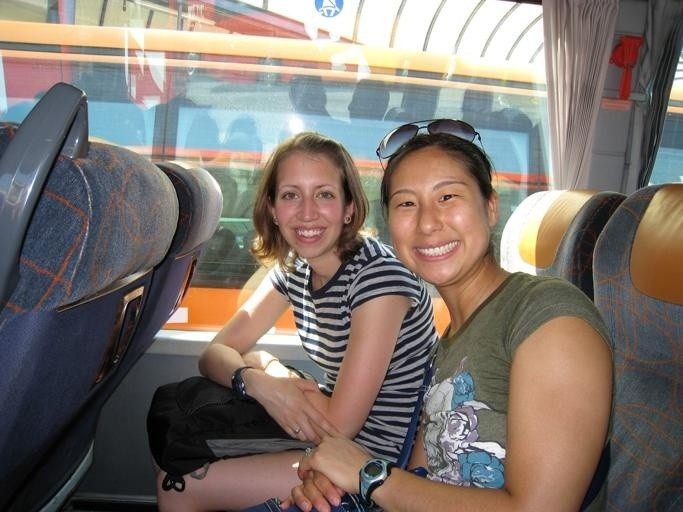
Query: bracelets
[[262, 358, 278, 372]]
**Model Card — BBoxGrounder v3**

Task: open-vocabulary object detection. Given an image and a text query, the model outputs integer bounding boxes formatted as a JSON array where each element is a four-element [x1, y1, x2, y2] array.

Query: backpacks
[[146, 364, 322, 491]]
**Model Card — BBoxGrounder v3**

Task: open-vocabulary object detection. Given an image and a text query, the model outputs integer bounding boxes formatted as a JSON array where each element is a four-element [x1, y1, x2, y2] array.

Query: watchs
[[230, 365, 254, 399], [357, 458, 400, 510]]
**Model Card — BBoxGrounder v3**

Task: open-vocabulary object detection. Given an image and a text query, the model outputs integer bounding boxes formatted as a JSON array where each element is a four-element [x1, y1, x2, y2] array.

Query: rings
[[305, 448, 312, 456], [294, 427, 301, 434]]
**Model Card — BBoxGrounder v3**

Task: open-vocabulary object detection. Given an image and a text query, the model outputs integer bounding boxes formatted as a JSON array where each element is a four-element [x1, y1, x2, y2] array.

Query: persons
[[252, 119, 614, 512], [233, 74, 520, 224], [153, 129, 438, 511], [184, 117, 221, 150]]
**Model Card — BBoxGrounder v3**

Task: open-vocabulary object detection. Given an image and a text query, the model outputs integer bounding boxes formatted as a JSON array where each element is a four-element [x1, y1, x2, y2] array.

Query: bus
[[0, 21, 683, 346]]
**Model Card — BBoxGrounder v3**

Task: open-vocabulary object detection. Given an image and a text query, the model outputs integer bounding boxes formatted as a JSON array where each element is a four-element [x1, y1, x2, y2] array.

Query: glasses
[[375, 119, 486, 171]]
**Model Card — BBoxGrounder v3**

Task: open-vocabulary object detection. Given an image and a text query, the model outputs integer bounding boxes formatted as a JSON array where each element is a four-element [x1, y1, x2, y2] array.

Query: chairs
[[0, 83, 180, 511], [5, 163, 223, 512], [582, 184, 682, 511], [501, 189, 627, 302]]
